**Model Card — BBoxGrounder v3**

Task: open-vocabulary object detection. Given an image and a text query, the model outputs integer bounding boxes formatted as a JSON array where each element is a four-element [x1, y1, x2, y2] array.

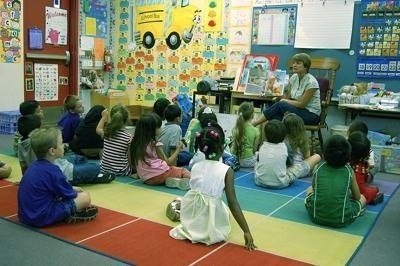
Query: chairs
[[288, 57, 340, 153]]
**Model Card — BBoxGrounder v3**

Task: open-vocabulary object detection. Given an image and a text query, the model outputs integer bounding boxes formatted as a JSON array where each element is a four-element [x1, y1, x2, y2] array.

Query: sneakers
[[372, 193, 384, 205], [174, 200, 181, 210], [166, 202, 181, 221], [165, 177, 190, 190], [62, 206, 98, 225], [233, 162, 241, 171], [93, 173, 116, 184]]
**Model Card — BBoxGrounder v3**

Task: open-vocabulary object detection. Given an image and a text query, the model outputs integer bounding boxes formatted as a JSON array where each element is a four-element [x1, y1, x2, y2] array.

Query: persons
[[18, 127, 98, 227], [11, 100, 45, 158], [96, 104, 139, 180], [190, 108, 204, 158], [152, 98, 171, 130], [166, 121, 258, 253], [230, 101, 261, 167], [69, 105, 104, 159], [0, 161, 12, 179], [158, 104, 193, 167], [345, 132, 384, 206], [253, 118, 303, 189], [16, 115, 116, 187], [184, 107, 207, 152], [57, 95, 85, 143], [133, 111, 191, 191], [190, 112, 236, 169], [345, 120, 379, 181], [282, 112, 321, 179], [250, 52, 321, 143], [304, 135, 367, 229]]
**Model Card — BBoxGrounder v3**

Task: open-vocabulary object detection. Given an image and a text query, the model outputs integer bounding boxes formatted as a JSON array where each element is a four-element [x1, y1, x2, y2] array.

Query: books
[[243, 67, 286, 96]]
